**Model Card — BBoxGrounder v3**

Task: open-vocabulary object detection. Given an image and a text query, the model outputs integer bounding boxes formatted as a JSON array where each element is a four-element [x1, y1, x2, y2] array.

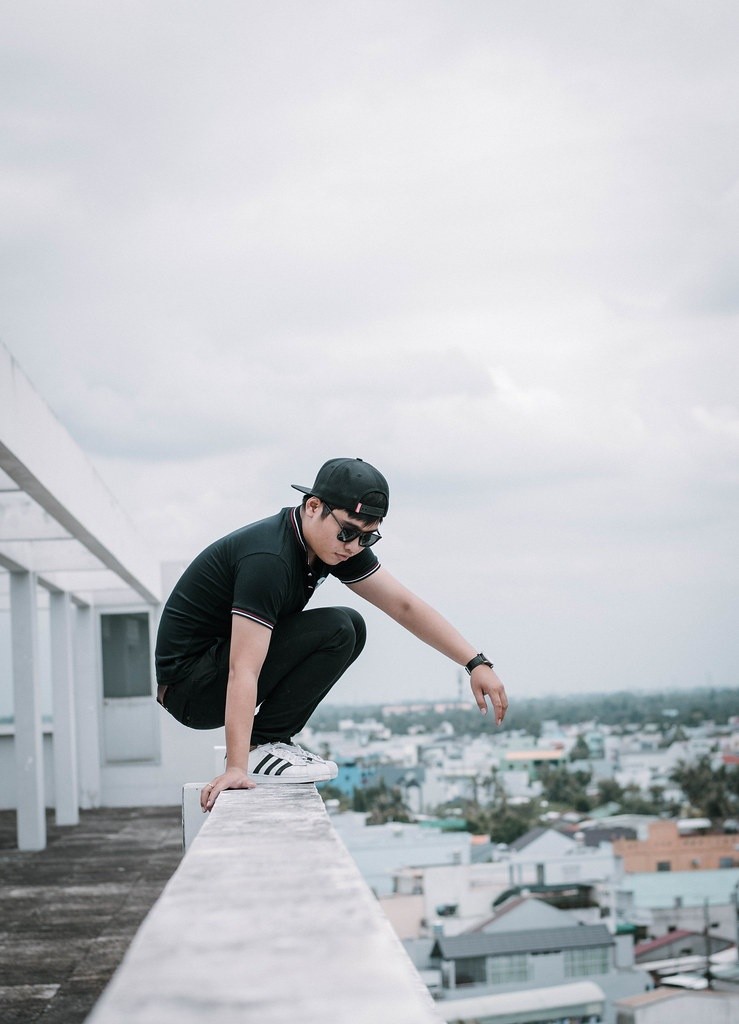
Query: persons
[[155, 457, 509, 814]]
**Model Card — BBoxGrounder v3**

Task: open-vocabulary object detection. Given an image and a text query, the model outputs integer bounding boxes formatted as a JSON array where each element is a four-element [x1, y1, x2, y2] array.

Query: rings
[[208, 784, 215, 789]]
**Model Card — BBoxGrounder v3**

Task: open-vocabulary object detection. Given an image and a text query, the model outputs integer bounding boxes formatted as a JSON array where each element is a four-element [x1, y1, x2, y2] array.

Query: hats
[[289, 457, 389, 516]]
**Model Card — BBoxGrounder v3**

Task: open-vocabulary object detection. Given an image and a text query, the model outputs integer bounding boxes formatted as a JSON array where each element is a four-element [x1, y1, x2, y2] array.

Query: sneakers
[[225, 744, 329, 784], [278, 742, 338, 779]]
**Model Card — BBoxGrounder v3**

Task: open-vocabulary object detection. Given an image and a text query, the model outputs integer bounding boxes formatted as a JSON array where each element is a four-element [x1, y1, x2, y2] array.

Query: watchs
[[464, 653, 493, 676]]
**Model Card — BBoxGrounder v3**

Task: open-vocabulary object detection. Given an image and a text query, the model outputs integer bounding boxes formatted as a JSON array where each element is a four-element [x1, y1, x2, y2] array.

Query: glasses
[[323, 502, 382, 548]]
